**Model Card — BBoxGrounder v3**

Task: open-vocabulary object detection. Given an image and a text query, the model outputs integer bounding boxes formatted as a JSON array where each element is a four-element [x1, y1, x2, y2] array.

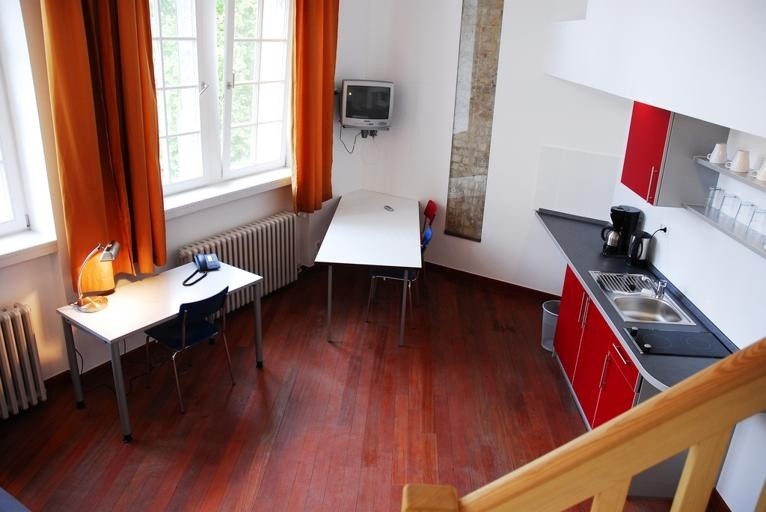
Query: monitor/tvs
[[342, 79, 394, 128]]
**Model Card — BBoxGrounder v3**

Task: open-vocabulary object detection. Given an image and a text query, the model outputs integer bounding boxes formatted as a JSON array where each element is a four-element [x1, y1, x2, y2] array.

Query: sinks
[[611, 295, 685, 324], [592, 272, 657, 295]]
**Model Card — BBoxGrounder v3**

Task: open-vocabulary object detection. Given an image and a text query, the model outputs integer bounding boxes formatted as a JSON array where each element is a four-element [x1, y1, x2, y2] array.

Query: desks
[[313, 188, 423, 348], [56, 262, 264, 443]]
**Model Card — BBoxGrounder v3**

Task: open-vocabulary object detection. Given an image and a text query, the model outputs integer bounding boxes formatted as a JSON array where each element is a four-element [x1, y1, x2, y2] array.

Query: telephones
[[193, 253, 220, 271]]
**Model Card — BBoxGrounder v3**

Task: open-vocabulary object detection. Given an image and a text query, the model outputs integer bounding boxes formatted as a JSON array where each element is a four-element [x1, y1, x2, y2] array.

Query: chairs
[[144, 286, 236, 416], [365, 200, 438, 325]]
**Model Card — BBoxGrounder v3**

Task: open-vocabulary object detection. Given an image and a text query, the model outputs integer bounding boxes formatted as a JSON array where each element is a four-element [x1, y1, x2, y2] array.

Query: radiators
[[176, 209, 298, 322], [0, 302, 47, 420]]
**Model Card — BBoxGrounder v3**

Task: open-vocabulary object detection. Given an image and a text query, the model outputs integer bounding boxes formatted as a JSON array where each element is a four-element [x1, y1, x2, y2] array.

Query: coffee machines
[[600, 205, 641, 258]]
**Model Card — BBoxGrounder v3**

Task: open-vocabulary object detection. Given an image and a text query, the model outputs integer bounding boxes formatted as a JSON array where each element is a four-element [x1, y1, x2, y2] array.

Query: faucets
[[641, 274, 668, 298]]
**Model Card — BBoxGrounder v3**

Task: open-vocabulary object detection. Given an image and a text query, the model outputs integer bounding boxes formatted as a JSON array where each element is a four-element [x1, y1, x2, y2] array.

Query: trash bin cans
[[541, 300, 560, 352]]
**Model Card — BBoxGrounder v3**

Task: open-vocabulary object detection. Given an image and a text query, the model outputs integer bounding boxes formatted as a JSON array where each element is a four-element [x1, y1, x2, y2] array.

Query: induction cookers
[[622, 326, 731, 359]]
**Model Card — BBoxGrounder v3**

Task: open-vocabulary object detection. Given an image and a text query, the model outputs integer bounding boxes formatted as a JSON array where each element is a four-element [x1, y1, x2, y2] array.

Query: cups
[[707, 143, 729, 164], [724, 150, 751, 173], [750, 159, 766, 182]]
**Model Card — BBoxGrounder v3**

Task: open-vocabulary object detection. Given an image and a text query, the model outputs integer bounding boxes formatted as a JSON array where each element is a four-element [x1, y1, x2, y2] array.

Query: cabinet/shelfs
[[684, 153, 766, 261], [554, 264, 590, 385], [571, 301, 640, 431], [620, 100, 730, 206]]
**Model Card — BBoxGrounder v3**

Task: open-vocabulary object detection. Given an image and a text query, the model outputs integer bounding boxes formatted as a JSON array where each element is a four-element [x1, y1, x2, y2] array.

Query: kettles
[[624, 229, 652, 270]]
